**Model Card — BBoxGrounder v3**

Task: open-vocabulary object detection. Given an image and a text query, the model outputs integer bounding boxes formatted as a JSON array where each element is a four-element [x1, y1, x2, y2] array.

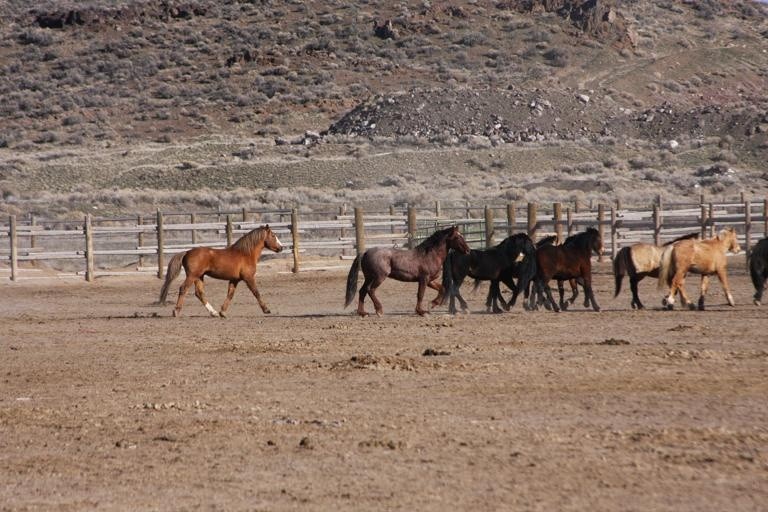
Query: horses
[[746, 237, 768, 306], [343, 224, 605, 318], [157, 224, 284, 319], [614, 225, 742, 311]]
